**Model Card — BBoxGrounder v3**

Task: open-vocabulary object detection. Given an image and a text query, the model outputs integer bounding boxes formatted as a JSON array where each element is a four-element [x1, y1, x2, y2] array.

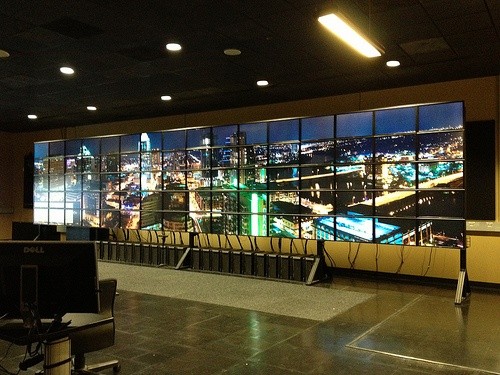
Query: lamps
[[317, 7, 386, 59]]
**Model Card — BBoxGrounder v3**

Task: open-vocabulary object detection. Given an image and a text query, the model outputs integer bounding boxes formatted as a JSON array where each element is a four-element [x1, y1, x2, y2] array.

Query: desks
[[0, 314, 114, 375]]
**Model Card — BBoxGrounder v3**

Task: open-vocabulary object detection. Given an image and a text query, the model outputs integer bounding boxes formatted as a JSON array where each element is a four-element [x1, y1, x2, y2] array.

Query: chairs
[[42, 278, 121, 375]]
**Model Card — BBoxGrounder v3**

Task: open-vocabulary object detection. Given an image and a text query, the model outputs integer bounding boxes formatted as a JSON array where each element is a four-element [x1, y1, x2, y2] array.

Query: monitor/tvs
[[1, 239, 101, 320], [13, 222, 102, 241]]
